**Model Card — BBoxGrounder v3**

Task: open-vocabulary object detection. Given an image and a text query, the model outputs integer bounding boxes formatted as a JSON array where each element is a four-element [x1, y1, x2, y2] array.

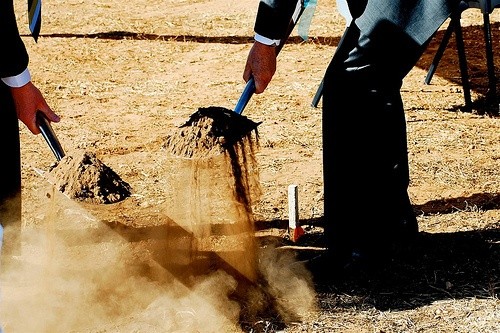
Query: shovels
[[156, 2, 308, 159], [30, 113, 132, 205]]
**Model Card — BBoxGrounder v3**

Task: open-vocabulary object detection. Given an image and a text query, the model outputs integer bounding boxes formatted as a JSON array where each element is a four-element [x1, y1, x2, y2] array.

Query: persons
[[242, 0, 472, 287], [0, 0, 61, 268]]
